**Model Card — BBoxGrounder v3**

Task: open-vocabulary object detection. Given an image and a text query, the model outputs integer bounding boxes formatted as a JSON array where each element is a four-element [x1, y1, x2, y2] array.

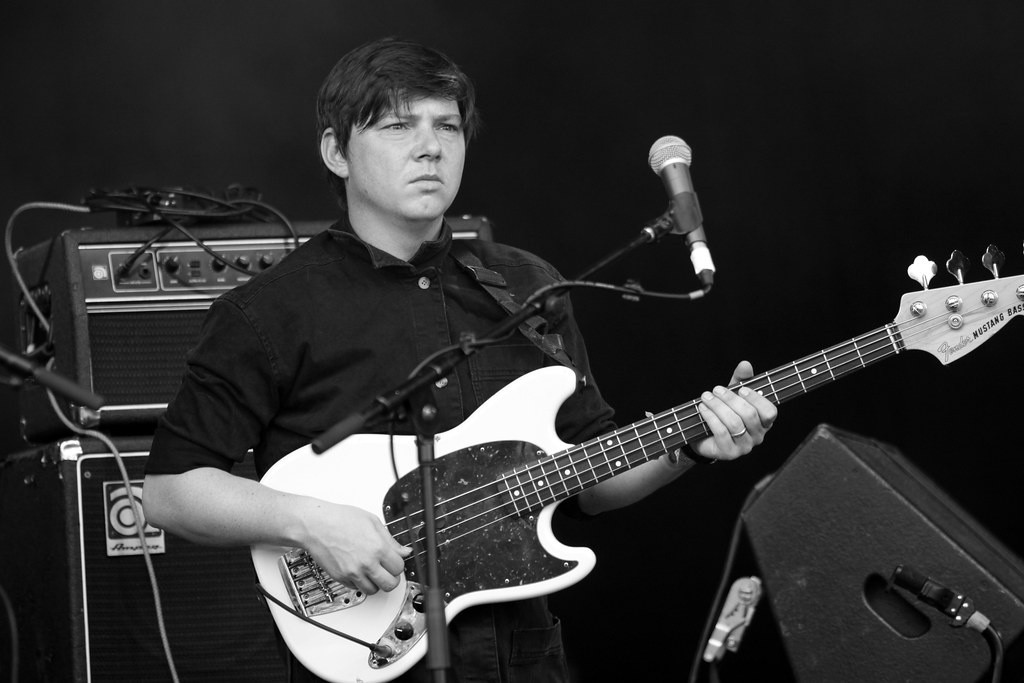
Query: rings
[[731, 428, 746, 437]]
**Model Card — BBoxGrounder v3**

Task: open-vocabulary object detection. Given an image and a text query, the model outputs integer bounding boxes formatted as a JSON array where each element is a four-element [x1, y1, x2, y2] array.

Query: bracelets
[[682, 444, 716, 465]]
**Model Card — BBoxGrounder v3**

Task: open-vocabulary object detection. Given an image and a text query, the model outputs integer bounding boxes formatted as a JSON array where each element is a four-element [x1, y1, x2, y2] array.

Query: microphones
[[647, 136, 715, 287]]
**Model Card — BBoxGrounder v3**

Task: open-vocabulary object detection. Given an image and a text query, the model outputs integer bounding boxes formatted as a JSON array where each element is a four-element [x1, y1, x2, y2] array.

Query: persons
[[141, 37, 778, 683]]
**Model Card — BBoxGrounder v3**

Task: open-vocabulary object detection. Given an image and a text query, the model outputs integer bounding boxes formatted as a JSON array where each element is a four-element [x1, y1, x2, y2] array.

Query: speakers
[[0, 436, 323, 683], [741, 424, 1024, 683]]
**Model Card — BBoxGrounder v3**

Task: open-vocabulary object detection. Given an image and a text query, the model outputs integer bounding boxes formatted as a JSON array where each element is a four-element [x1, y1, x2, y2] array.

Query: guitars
[[247, 234, 1024, 683]]
